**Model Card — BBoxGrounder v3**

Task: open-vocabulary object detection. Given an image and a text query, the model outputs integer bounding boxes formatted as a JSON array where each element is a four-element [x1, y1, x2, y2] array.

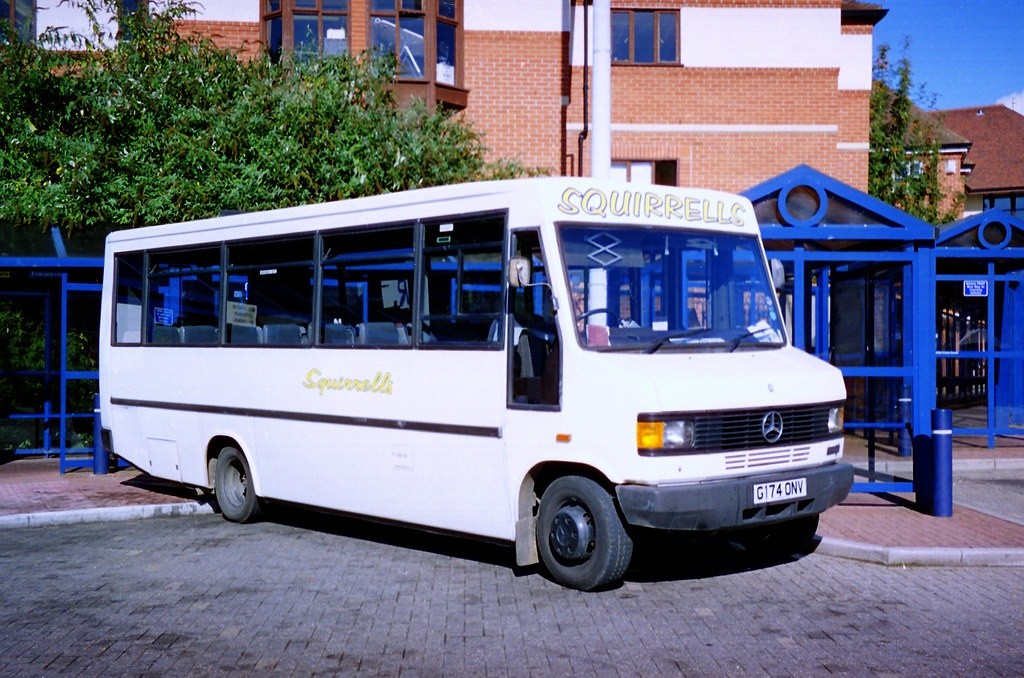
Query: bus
[[99, 176, 854, 594]]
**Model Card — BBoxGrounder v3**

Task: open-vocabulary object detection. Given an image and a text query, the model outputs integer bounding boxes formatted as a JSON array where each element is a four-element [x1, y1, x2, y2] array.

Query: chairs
[[146, 313, 500, 348]]
